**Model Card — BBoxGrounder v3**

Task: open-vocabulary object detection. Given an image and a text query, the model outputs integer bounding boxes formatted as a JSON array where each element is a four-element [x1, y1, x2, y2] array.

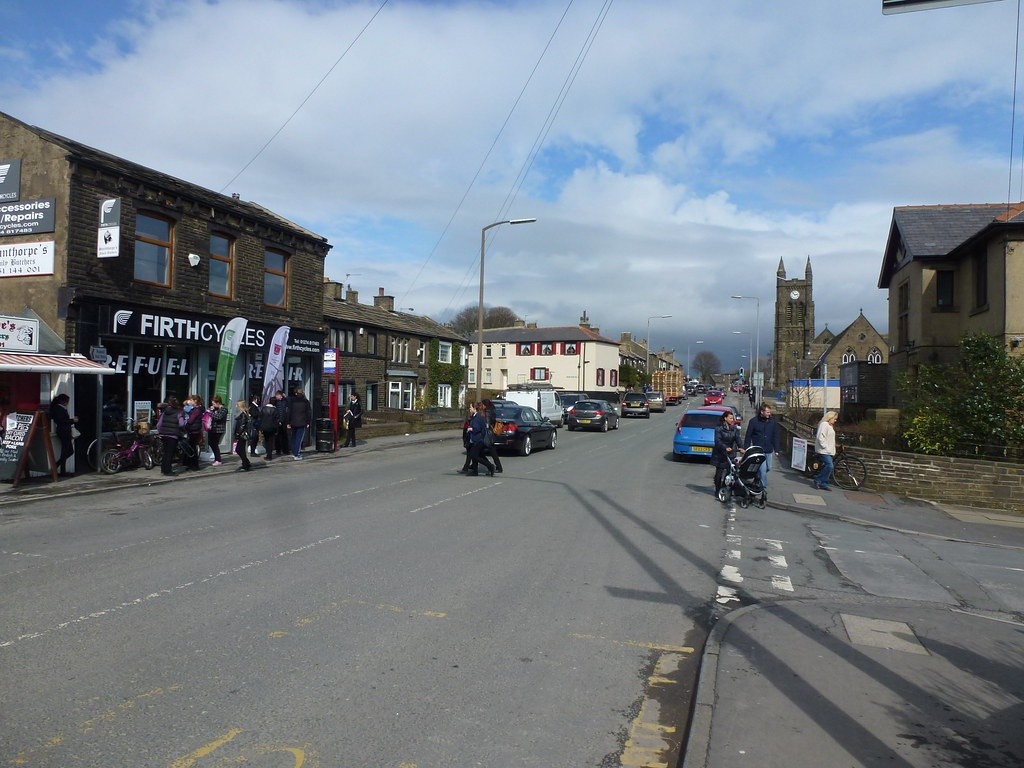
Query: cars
[[567, 399, 619, 432], [686, 384, 715, 396], [560, 393, 590, 425], [462, 399, 557, 456], [673, 405, 743, 464], [704, 380, 749, 405]]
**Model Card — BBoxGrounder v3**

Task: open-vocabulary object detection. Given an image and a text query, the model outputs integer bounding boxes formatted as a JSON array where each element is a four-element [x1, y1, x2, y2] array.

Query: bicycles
[[804, 433, 871, 491], [85, 422, 163, 475]]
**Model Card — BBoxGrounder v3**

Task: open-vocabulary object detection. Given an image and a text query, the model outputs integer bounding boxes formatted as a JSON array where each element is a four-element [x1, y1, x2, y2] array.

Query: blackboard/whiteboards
[[0, 409, 55, 478]]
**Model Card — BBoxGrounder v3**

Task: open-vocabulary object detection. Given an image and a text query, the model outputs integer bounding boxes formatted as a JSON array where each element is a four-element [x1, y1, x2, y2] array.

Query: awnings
[[0, 354, 115, 374]]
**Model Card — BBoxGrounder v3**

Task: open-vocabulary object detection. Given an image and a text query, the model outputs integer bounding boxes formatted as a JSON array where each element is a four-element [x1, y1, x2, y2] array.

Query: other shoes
[[457, 469, 468, 474], [343, 443, 348, 447], [292, 455, 303, 461], [490, 464, 495, 477], [494, 468, 502, 473], [282, 450, 290, 455], [349, 444, 356, 447], [237, 456, 251, 472], [186, 463, 200, 471], [264, 455, 272, 461], [465, 470, 478, 476], [814, 479, 832, 491], [161, 469, 178, 476], [276, 452, 281, 455]]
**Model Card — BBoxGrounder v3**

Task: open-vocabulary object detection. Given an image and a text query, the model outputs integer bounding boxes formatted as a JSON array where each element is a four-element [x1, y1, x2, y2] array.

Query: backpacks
[[490, 420, 504, 436], [240, 411, 258, 441], [480, 427, 493, 448]]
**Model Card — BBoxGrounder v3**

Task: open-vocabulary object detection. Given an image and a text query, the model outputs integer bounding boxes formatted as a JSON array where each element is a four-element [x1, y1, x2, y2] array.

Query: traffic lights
[[740, 369, 743, 376]]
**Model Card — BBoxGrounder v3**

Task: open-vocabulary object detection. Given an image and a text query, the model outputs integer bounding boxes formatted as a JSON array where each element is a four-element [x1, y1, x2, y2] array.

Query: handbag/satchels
[[70, 424, 81, 439]]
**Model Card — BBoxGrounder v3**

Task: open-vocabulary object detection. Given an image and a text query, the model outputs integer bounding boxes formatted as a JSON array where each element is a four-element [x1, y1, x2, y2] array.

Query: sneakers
[[209, 460, 222, 466]]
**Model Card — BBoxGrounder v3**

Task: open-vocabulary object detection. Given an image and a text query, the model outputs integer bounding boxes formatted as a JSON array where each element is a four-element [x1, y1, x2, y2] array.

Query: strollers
[[718, 446, 772, 510]]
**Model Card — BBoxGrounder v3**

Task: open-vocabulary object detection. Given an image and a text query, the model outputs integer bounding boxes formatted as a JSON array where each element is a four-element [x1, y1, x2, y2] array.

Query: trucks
[[652, 370, 686, 405]]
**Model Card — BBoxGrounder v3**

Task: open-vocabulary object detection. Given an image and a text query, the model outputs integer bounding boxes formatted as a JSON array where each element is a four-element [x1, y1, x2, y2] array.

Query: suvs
[[621, 392, 652, 418], [646, 391, 667, 413]]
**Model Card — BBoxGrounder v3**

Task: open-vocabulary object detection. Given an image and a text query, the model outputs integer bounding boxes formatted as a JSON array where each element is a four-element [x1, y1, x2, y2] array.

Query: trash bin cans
[[315, 417, 334, 454]]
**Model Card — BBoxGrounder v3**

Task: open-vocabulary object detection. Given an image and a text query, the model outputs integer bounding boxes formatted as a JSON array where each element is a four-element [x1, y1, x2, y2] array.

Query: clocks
[[789, 290, 799, 299]]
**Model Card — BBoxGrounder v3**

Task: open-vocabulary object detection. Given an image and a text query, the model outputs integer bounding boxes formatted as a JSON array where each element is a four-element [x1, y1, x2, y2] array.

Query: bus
[[689, 381, 699, 387]]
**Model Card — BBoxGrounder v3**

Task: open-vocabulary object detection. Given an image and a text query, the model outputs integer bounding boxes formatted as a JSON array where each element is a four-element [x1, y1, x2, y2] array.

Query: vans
[[506, 391, 565, 428]]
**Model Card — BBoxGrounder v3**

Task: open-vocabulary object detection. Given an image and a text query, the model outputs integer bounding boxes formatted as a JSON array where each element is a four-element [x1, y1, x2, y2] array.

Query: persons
[[340, 392, 364, 448], [749, 392, 754, 408], [710, 411, 745, 498], [457, 399, 503, 477], [206, 395, 228, 466], [104, 393, 125, 412], [234, 400, 252, 472], [813, 411, 838, 491], [744, 404, 780, 509], [157, 395, 206, 476], [249, 386, 311, 461], [48, 393, 78, 477]]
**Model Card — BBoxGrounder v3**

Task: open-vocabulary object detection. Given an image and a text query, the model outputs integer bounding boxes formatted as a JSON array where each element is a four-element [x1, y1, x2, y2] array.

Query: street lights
[[731, 296, 759, 409], [475, 216, 537, 404], [793, 349, 799, 379], [687, 340, 704, 381], [646, 315, 673, 374]]
